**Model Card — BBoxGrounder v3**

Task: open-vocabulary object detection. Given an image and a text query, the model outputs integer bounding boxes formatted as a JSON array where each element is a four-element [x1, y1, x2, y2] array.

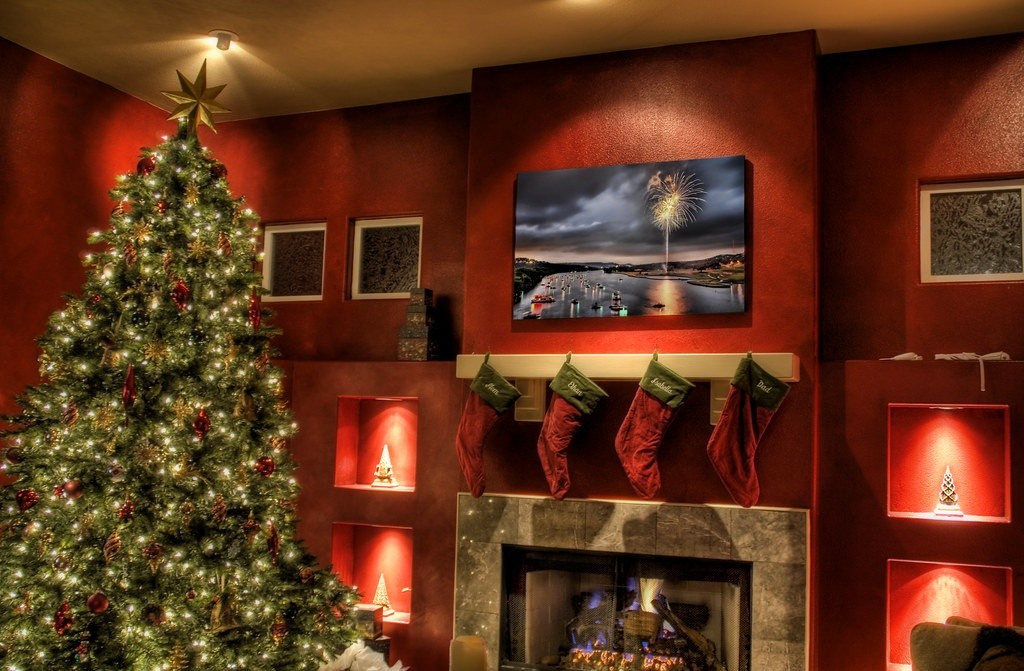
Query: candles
[[449, 635, 487, 671]]
